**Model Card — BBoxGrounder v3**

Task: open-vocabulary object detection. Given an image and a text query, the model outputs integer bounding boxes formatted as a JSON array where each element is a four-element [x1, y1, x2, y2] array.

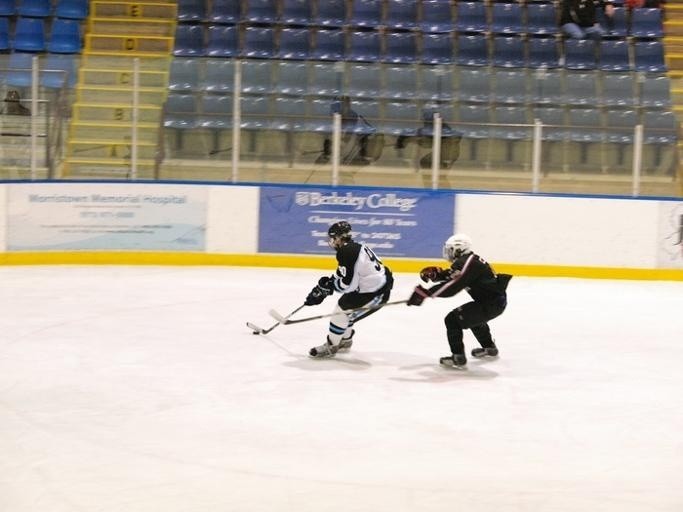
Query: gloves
[[407, 284, 428, 307], [303, 274, 336, 306], [420, 266, 444, 283]]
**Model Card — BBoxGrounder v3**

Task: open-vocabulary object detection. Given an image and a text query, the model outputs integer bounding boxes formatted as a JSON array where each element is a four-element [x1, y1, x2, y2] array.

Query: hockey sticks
[[246, 302, 305, 335], [271, 300, 407, 326]]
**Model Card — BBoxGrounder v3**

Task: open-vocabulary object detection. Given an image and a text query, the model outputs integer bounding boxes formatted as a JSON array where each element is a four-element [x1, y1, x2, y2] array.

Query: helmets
[[443, 233, 473, 261], [328, 220, 352, 249]]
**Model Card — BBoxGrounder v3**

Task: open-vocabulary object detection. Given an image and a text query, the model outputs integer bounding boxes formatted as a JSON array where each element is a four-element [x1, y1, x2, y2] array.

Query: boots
[[329, 328, 355, 351], [310, 335, 339, 356], [471, 344, 498, 357], [439, 353, 467, 366]]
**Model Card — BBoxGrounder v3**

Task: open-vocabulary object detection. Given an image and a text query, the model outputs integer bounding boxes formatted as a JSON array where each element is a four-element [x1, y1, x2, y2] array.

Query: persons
[[304, 221, 393, 357], [0, 90, 31, 115], [406, 235, 512, 367], [395, 108, 462, 188], [315, 95, 385, 185], [559, 0, 616, 65]]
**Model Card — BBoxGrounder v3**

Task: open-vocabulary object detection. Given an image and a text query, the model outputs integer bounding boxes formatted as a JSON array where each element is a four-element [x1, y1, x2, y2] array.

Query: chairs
[[2, 2, 90, 179], [158, 3, 679, 177]]
